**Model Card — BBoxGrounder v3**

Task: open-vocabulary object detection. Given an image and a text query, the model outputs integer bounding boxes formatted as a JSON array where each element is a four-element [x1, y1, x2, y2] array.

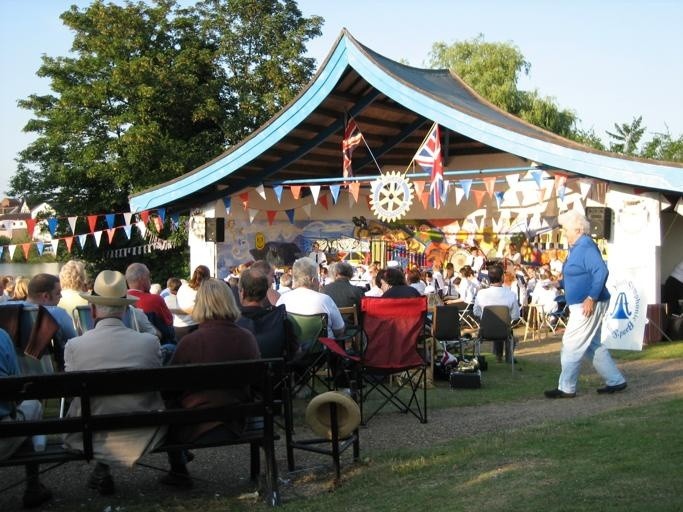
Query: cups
[[32, 434, 47, 452]]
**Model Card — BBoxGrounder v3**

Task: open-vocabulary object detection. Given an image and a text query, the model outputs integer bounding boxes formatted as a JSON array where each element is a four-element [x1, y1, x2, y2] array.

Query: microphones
[[320, 260, 326, 265]]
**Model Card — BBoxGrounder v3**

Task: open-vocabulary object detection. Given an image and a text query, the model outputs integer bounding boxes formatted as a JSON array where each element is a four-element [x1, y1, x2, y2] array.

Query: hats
[[306, 392, 361, 439], [79, 270, 139, 306], [237, 264, 247, 275]]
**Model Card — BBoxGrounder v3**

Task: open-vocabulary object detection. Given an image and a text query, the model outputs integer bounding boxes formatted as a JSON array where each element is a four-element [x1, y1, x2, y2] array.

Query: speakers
[[205, 218, 224, 242], [585, 207, 611, 239]]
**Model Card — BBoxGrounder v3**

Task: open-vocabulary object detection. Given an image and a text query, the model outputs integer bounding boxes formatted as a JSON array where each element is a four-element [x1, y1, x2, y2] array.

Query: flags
[[414, 123, 443, 208], [343, 108, 362, 188]]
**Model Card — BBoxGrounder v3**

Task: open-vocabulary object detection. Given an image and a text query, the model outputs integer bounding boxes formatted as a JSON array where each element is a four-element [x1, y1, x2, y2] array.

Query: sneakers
[[160, 471, 194, 488], [544, 389, 575, 398], [79, 474, 111, 493], [597, 382, 626, 393], [24, 483, 51, 506]]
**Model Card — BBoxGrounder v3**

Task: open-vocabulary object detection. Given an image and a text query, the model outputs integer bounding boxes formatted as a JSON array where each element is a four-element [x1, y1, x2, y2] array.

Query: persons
[[163, 279, 262, 487], [0, 243, 563, 399], [545, 211, 627, 397], [59, 270, 167, 489], [0, 328, 52, 508]]
